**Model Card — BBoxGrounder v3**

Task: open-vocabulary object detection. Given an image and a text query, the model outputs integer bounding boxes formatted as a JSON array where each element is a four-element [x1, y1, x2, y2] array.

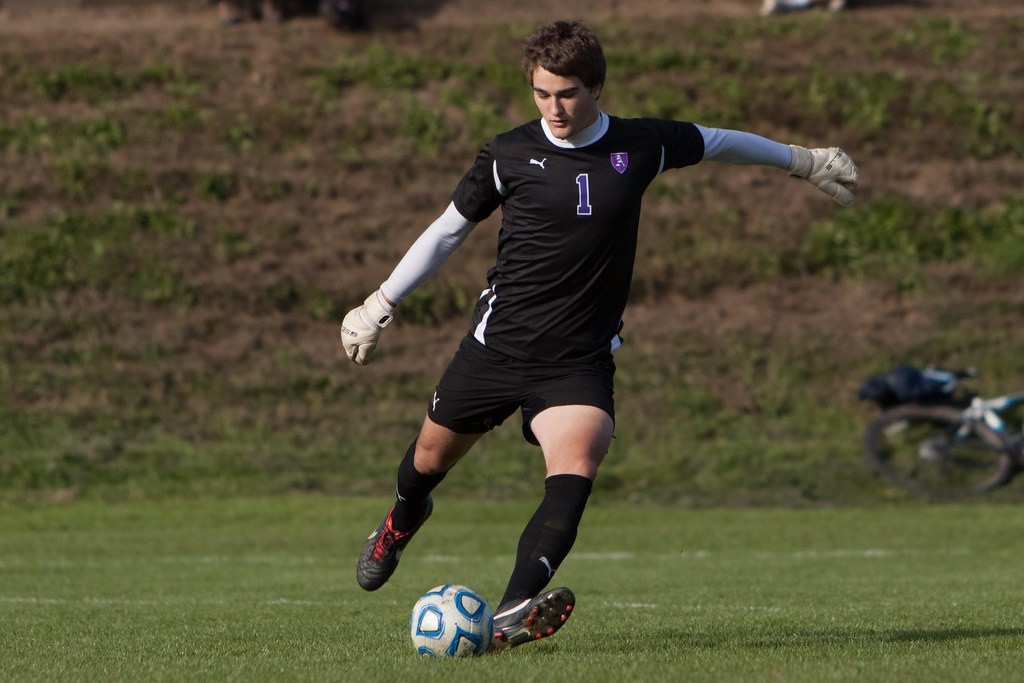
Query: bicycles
[[857, 364, 1023, 499]]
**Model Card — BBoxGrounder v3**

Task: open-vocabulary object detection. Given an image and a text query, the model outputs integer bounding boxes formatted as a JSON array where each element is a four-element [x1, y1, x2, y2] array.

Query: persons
[[341, 19, 856, 654]]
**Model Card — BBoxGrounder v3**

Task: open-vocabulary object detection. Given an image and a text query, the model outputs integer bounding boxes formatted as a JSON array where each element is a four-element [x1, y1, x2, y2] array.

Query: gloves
[[788, 143, 858, 207], [341, 291, 396, 365]]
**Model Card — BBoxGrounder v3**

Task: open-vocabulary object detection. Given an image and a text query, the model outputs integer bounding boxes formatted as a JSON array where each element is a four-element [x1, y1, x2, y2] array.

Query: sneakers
[[490, 587, 576, 654], [356, 494, 433, 592]]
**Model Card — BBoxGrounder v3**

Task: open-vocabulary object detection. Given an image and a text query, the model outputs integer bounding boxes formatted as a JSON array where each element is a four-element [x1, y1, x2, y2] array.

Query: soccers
[[410, 582, 492, 661]]
